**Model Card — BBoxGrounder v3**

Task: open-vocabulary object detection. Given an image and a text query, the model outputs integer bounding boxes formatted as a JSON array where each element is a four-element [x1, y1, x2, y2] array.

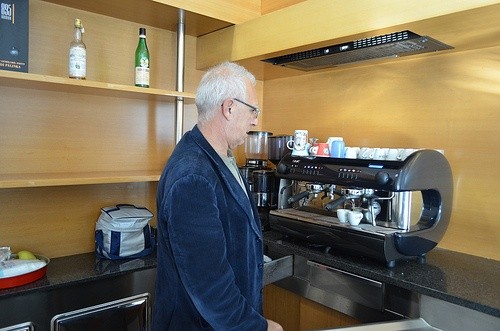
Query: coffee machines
[[267, 136, 291, 169], [237, 131, 273, 192]]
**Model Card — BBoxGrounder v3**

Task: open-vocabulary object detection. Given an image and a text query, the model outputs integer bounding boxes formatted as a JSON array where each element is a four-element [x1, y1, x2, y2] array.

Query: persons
[[151, 60, 283, 331]]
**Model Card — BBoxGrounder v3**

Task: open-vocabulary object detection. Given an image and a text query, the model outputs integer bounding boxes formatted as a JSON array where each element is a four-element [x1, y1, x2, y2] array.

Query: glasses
[[234, 97, 260, 120]]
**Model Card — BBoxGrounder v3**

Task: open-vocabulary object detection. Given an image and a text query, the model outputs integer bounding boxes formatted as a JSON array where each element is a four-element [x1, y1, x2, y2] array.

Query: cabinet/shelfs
[[0, 267, 158, 331], [0, 70, 196, 260]]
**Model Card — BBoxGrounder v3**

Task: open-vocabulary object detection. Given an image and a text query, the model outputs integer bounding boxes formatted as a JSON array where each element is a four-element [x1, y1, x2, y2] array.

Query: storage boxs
[[0, 253, 51, 290]]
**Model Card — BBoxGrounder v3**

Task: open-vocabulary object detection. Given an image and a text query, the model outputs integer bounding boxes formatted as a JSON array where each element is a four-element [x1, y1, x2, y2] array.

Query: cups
[[345, 147, 445, 161], [286, 129, 309, 156], [336, 208, 350, 222], [308, 142, 331, 156], [348, 211, 363, 225], [326, 136, 345, 158]]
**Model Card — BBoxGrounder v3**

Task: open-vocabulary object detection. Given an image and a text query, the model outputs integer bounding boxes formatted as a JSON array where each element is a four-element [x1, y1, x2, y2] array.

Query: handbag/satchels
[[95, 203, 157, 262]]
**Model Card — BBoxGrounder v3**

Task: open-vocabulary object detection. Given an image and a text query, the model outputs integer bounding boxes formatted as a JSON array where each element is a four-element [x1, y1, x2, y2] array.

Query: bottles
[[68, 18, 87, 79], [135, 27, 150, 87]]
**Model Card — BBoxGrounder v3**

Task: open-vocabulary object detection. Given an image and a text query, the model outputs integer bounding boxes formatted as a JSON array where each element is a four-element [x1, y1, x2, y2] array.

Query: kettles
[[253, 170, 277, 208]]
